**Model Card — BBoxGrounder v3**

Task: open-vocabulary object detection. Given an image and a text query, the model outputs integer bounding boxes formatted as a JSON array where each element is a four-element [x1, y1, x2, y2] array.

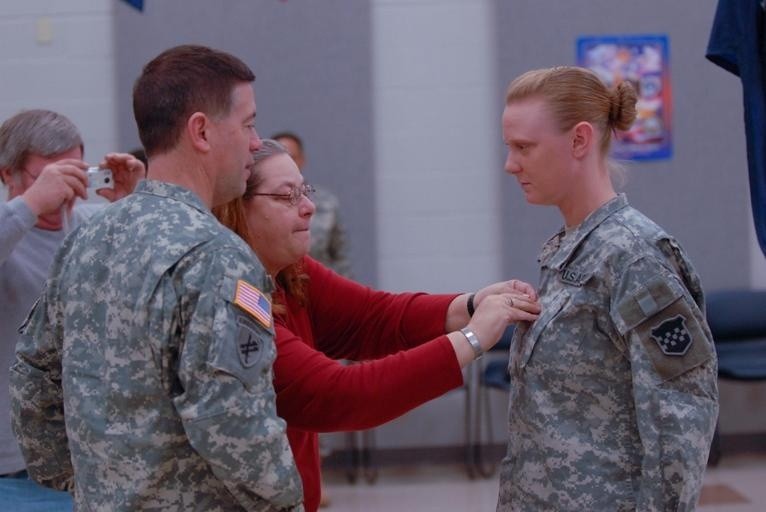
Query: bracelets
[[462, 326, 485, 364], [467, 292, 479, 321]]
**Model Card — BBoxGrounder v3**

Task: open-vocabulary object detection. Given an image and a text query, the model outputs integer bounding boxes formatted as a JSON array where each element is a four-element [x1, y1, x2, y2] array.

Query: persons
[[495, 67, 718, 510], [211, 139, 541, 512], [268, 133, 351, 507], [8, 46, 306, 511], [0, 109, 149, 512]]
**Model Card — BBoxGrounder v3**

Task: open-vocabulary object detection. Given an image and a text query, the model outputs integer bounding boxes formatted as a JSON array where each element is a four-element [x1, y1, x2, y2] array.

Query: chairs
[[365, 353, 477, 488], [705, 286, 766, 464], [477, 322, 514, 478]]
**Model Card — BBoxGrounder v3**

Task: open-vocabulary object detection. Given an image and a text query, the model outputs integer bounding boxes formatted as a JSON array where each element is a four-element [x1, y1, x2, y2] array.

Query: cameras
[[83, 167, 115, 189]]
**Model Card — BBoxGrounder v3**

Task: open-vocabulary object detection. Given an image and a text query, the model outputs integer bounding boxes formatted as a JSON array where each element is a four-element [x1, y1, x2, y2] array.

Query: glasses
[[244, 182, 317, 208]]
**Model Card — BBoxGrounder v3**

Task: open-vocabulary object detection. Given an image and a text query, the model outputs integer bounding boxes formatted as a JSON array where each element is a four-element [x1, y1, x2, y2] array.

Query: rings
[[507, 298, 515, 307]]
